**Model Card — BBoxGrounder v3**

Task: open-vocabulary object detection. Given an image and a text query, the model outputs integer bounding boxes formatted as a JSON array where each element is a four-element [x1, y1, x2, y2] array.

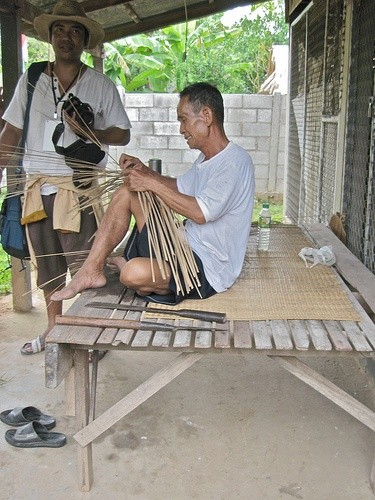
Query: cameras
[[62, 96, 95, 141]]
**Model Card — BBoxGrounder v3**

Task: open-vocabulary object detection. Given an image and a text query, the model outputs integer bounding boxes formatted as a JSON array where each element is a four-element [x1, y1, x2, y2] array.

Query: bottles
[[257, 202, 271, 252]]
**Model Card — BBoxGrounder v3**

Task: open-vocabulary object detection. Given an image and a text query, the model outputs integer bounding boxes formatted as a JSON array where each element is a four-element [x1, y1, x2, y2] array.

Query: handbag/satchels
[[0, 190, 29, 259]]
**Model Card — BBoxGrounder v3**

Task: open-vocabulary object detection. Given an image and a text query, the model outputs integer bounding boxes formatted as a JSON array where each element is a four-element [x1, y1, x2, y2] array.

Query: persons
[[48, 83, 255, 301], [0, 0, 132, 364]]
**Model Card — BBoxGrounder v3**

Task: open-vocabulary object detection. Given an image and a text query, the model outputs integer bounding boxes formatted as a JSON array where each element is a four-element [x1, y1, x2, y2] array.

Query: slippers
[[20, 335, 46, 355], [89, 349, 107, 362], [5, 421, 66, 448], [0, 406, 56, 429]]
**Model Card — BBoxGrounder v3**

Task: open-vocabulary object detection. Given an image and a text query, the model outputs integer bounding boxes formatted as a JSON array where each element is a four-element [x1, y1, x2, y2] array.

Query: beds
[[44, 222, 373, 492]]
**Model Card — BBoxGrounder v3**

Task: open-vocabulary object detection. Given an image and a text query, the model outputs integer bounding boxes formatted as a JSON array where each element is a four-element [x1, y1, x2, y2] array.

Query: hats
[[34, 0, 105, 50]]
